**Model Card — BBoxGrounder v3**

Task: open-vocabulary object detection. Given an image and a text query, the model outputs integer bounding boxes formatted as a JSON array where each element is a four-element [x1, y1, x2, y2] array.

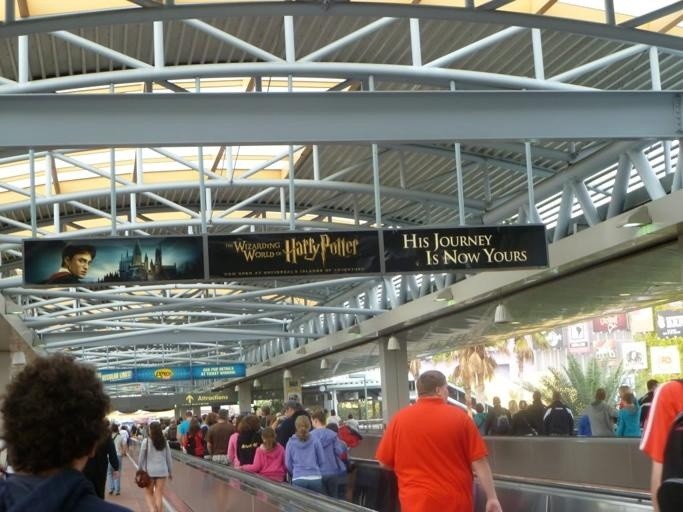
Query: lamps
[[5, 300, 23, 314], [618, 205, 653, 230], [233, 286, 512, 393], [9, 348, 27, 366]]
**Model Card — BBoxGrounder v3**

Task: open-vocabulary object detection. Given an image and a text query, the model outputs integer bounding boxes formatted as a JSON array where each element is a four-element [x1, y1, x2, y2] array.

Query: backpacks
[[184, 431, 197, 454], [491, 408, 508, 433]]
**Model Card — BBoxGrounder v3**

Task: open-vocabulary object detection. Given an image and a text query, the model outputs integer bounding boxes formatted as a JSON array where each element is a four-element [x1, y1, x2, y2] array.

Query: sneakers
[[108, 488, 121, 496]]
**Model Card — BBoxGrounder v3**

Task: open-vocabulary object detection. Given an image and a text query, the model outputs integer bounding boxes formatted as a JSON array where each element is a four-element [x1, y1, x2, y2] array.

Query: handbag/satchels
[[135, 471, 151, 489]]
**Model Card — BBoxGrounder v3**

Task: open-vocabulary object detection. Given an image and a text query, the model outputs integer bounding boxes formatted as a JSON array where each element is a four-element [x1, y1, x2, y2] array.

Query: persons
[[639, 378, 682, 511], [136, 421, 173, 512], [1, 351, 131, 511], [466, 377, 659, 439], [35, 244, 96, 284], [89, 399, 366, 496], [375, 369, 503, 511]]
